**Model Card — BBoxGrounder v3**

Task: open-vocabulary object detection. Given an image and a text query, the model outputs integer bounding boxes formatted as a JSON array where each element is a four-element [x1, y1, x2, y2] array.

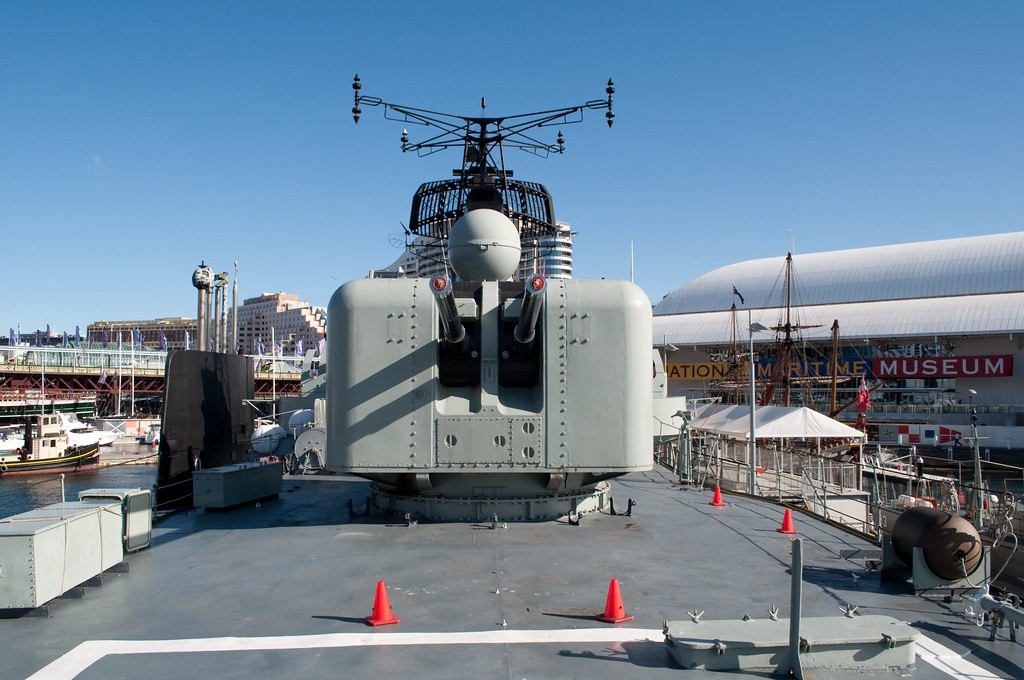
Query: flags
[[8, 322, 326, 359], [733, 285, 744, 304], [856, 380, 871, 412]]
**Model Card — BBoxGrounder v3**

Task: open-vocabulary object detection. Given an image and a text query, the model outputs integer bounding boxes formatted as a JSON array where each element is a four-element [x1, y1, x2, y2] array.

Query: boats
[[0, 78, 1024, 680]]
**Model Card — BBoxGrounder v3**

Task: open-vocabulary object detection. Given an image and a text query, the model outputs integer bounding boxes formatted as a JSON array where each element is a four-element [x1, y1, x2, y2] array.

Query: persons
[[954, 432, 962, 446]]
